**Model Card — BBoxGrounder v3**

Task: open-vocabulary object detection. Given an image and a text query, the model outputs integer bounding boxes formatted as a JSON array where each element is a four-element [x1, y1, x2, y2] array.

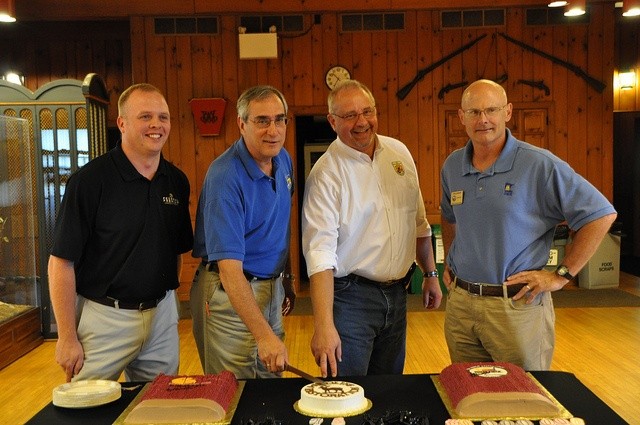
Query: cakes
[[300, 380, 365, 413]]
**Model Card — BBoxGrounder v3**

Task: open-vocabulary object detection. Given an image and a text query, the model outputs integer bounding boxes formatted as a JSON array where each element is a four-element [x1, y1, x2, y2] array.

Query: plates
[[430, 372, 574, 420], [52, 380, 121, 409], [111, 380, 248, 425], [293, 398, 373, 418]]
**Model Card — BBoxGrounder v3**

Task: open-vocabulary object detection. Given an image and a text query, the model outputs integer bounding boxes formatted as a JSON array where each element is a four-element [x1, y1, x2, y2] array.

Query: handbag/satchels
[[579, 229, 626, 290]]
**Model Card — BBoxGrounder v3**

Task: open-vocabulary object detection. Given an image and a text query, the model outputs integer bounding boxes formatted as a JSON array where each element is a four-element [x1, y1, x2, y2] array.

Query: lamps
[[564, 0, 587, 16], [617, 68, 636, 91], [620, 0, 640, 17], [546, 0, 569, 8], [1, 0, 18, 24]]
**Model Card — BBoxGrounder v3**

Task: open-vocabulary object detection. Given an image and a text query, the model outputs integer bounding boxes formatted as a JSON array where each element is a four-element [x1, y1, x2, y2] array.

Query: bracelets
[[421, 270, 439, 277]]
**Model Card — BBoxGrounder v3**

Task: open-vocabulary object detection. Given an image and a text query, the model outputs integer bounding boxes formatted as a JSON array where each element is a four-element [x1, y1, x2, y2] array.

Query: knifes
[[284, 361, 326, 387]]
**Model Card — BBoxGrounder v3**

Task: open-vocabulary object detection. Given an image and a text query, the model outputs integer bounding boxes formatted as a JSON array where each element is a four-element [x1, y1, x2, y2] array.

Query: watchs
[[556, 264, 574, 280]]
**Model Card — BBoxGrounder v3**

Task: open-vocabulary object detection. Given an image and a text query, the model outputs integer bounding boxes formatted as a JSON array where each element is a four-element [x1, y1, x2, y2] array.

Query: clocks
[[324, 65, 352, 92]]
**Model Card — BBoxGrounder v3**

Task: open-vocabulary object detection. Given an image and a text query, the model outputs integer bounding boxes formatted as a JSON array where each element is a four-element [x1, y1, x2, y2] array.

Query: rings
[[525, 285, 531, 290], [266, 364, 270, 368]]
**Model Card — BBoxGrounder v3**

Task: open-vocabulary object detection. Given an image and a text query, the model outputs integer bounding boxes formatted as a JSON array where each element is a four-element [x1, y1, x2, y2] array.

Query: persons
[[187, 85, 296, 379], [443, 80, 619, 371], [300, 79, 443, 379], [47, 82, 194, 382]]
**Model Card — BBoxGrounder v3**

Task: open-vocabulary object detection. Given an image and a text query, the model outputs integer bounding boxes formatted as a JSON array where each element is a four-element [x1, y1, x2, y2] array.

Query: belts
[[89, 291, 167, 311], [450, 274, 529, 298], [347, 262, 417, 289], [208, 261, 280, 282]]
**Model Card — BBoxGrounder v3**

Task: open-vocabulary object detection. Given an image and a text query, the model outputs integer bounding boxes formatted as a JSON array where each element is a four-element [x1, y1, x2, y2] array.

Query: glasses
[[247, 117, 289, 128], [333, 109, 376, 120], [463, 107, 504, 119]]
